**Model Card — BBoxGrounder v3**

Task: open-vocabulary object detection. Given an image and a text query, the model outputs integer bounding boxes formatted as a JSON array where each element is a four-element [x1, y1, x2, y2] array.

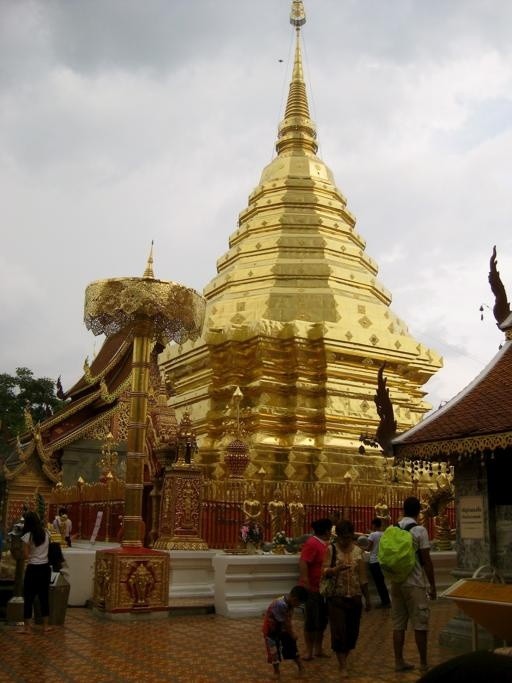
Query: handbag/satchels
[[319, 542, 338, 599], [64, 535, 72, 548], [47, 540, 66, 565]]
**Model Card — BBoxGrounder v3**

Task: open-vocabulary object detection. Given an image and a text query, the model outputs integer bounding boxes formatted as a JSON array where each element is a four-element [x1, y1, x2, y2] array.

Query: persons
[[261, 584, 309, 682], [55, 513, 68, 544], [319, 516, 372, 680], [178, 410, 195, 437], [376, 492, 392, 530], [357, 517, 394, 610], [297, 516, 332, 662], [52, 508, 72, 547], [381, 495, 438, 673], [289, 487, 308, 538], [12, 507, 53, 634], [268, 487, 286, 540], [242, 484, 265, 548]]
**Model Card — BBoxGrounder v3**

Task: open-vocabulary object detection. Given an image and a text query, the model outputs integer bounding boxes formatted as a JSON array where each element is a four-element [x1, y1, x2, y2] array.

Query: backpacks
[[377, 520, 420, 584]]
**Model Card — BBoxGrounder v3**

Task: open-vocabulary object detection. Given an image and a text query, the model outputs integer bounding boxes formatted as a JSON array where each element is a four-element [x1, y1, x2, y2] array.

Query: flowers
[[239, 524, 263, 543], [272, 531, 289, 545]]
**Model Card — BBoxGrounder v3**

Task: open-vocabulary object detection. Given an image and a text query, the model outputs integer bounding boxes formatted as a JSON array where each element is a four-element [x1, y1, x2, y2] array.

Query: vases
[[246, 540, 255, 554]]
